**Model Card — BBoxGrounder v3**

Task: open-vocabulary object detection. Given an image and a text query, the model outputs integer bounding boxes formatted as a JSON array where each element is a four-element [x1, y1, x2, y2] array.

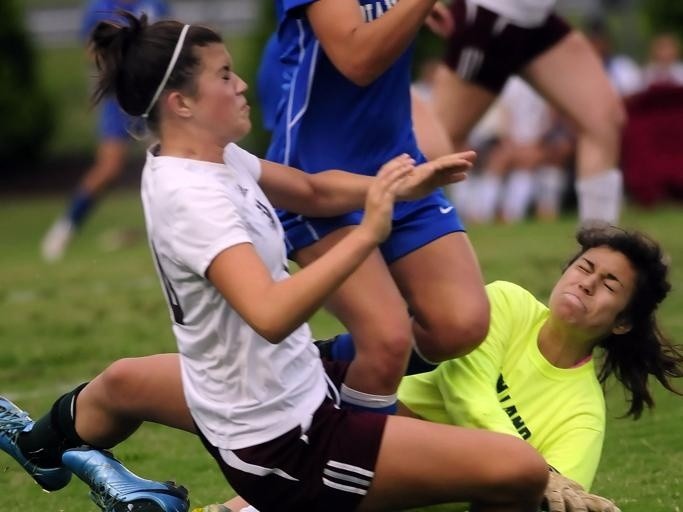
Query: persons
[[88, 10, 550, 511], [411, 0, 682, 236], [36, 0, 170, 261], [254, 1, 495, 417], [0, 220, 682, 511]]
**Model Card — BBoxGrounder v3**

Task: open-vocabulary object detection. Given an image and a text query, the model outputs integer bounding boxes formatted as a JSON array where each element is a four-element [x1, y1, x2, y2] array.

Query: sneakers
[[62, 446, 191, 512], [1, 396, 72, 492]]
